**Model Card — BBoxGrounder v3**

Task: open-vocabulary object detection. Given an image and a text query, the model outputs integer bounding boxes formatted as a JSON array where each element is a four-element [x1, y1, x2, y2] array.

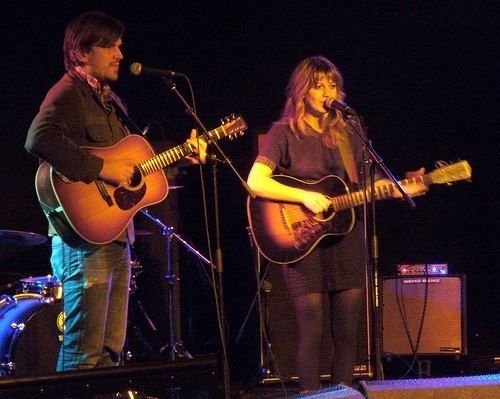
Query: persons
[[247, 56, 424, 393], [25, 13, 207, 371]]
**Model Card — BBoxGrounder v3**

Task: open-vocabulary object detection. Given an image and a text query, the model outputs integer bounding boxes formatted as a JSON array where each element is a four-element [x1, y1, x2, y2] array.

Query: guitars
[[246, 158, 474, 264], [34, 111, 250, 251]]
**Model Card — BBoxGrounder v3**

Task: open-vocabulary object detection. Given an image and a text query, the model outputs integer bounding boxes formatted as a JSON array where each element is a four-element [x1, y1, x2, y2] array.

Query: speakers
[[278, 385, 366, 399], [265, 260, 374, 381], [118, 185, 190, 361], [355, 372, 500, 399], [375, 276, 468, 357]]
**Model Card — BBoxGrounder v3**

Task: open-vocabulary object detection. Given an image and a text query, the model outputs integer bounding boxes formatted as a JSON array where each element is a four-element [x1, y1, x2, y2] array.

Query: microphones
[[130, 63, 184, 77], [326, 99, 355, 114]]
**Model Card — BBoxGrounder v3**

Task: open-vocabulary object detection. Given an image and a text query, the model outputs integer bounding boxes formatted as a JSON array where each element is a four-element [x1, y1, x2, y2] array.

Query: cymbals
[[0, 229, 48, 248]]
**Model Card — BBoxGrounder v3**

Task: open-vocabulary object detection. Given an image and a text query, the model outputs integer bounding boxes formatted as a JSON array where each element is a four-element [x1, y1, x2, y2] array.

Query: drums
[[0, 292, 64, 379], [13, 275, 64, 305]]
